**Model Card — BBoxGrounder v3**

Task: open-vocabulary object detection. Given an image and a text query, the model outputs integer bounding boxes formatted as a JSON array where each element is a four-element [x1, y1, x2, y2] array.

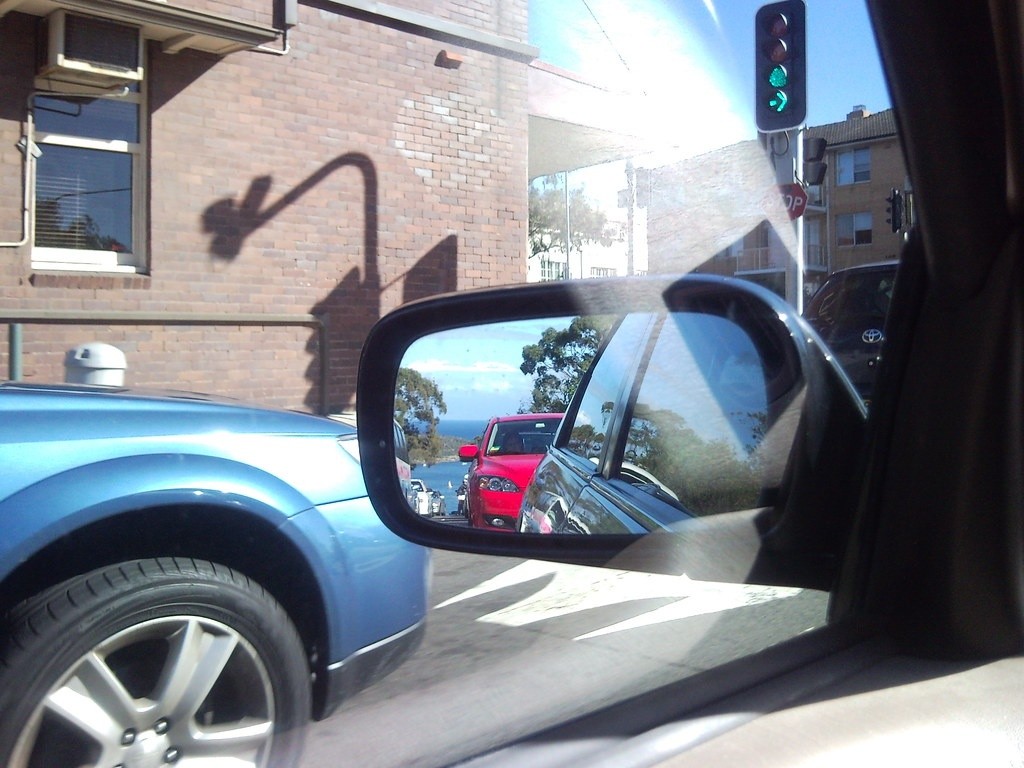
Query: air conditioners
[[37, 8, 144, 86]]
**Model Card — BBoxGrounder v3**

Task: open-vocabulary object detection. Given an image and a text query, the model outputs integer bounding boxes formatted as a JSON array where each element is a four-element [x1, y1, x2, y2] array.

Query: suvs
[[805, 261, 899, 401]]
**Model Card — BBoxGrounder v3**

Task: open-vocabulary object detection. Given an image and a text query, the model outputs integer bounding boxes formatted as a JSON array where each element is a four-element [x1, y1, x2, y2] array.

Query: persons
[[457, 481, 465, 512], [503, 431, 524, 454]]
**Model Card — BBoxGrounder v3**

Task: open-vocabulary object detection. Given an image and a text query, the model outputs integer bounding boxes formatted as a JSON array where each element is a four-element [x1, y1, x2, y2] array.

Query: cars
[[457, 412, 566, 531], [1, 380, 427, 768], [393, 421, 445, 516], [518, 308, 771, 534]]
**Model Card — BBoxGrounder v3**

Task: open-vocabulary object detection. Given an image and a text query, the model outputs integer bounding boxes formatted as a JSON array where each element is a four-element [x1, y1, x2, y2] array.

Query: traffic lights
[[757, 0, 808, 137], [886, 188, 904, 233]]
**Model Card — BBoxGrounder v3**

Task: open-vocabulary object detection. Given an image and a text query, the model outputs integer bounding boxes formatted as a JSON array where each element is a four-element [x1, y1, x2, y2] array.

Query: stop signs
[[770, 184, 807, 222]]
